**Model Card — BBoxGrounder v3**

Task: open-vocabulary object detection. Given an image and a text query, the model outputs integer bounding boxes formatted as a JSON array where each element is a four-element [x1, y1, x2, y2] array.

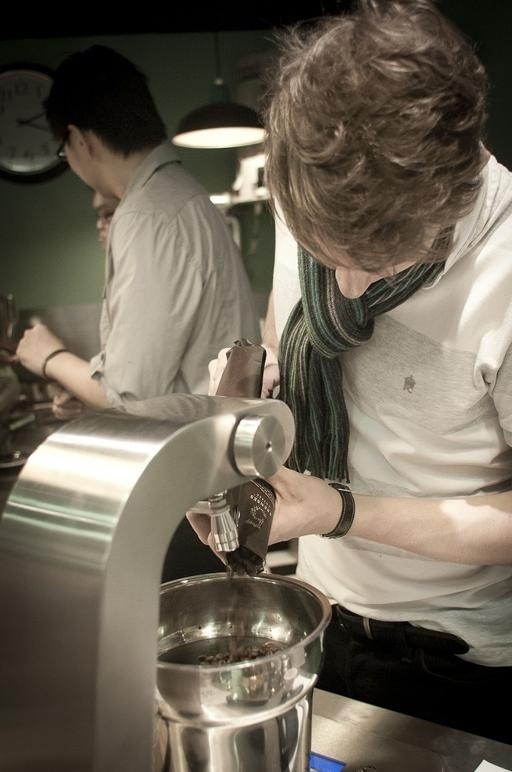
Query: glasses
[[55, 131, 73, 161]]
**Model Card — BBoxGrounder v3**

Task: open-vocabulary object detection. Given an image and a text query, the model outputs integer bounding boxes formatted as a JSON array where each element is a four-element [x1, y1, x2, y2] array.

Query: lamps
[[171, 32, 270, 150]]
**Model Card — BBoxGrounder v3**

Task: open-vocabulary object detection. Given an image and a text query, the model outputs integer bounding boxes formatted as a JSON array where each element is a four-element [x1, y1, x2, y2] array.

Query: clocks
[[0, 62, 69, 184]]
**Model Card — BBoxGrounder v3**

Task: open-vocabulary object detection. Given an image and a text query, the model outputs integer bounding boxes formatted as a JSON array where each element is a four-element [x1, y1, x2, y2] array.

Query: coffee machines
[[0, 392, 335, 772]]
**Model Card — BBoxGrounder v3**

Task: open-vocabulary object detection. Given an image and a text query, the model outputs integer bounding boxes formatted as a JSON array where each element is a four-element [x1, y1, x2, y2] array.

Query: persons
[[92, 193, 120, 253], [13, 60, 261, 422], [182, 0, 509, 745]]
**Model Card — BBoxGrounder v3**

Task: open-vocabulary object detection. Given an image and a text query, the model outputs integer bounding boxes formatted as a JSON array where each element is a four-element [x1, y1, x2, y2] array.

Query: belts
[[331, 602, 470, 661]]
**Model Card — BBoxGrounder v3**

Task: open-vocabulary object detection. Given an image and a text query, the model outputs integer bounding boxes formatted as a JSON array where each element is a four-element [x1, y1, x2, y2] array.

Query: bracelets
[[42, 348, 71, 380], [322, 482, 355, 540]]
